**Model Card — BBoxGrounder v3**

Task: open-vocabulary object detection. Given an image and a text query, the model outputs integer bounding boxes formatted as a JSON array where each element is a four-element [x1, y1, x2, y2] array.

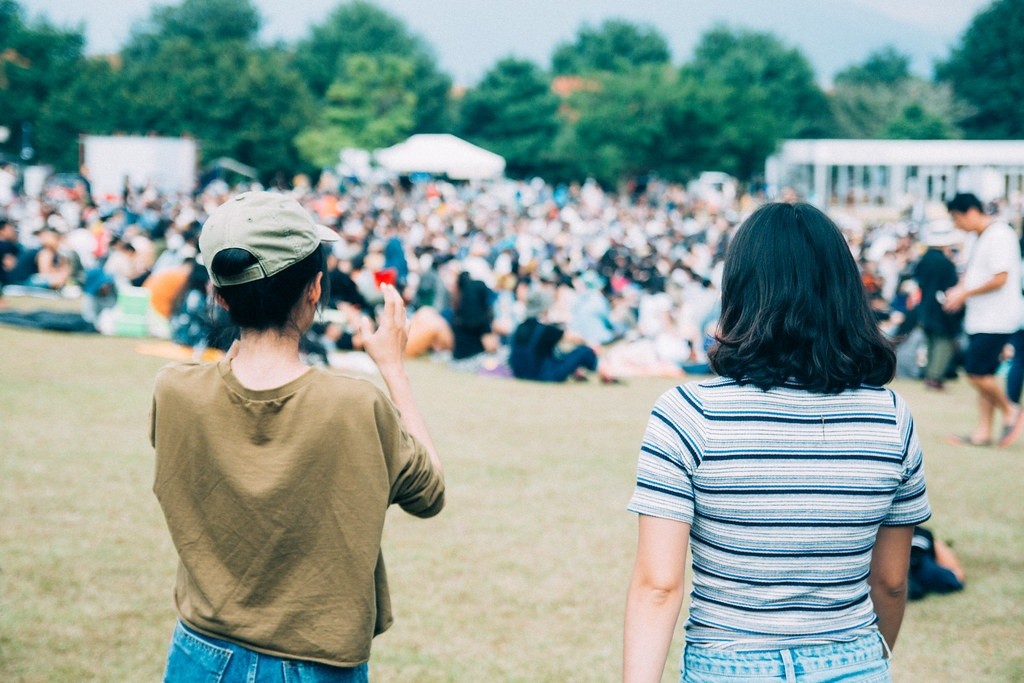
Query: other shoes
[[948, 435, 992, 449], [999, 411, 1024, 448]]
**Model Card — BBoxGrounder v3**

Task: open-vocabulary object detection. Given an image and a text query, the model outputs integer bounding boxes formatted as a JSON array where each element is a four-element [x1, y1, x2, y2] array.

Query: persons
[[912, 241, 964, 388], [1001, 206, 1024, 441], [622, 202, 930, 683], [909, 527, 964, 600], [144, 190, 447, 682], [941, 194, 1022, 446], [1, 158, 923, 385]]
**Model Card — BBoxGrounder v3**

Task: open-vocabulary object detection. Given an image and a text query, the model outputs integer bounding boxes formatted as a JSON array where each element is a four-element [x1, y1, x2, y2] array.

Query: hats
[[199, 191, 340, 287], [924, 222, 956, 246]]
